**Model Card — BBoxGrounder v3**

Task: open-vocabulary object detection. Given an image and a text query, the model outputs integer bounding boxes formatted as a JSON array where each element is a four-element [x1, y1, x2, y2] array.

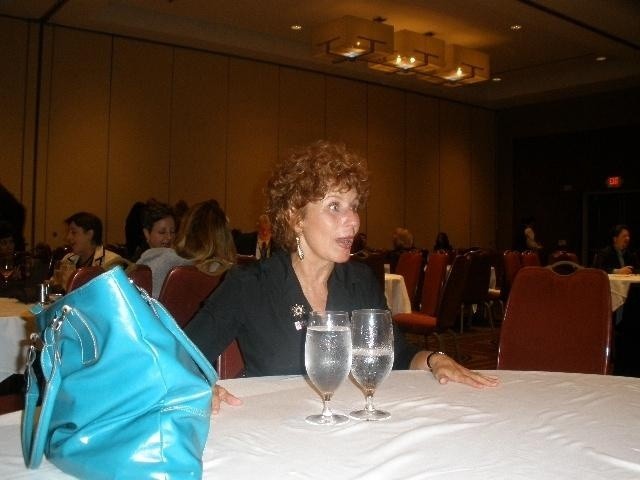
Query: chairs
[[64, 253, 258, 382], [386, 247, 611, 376]]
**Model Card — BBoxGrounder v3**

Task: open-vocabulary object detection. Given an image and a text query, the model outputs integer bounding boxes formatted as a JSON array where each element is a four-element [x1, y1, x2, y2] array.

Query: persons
[[600, 227, 640, 275], [1, 184, 280, 304], [382, 227, 415, 277], [184, 141, 499, 413], [545, 238, 579, 269], [434, 231, 455, 252]]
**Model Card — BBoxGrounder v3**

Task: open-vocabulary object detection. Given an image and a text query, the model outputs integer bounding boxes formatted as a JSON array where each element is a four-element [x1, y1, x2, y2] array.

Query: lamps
[[310, 14, 491, 89]]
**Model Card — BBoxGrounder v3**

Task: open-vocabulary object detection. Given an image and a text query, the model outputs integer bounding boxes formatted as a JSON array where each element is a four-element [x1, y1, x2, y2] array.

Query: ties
[[262, 242, 266, 259]]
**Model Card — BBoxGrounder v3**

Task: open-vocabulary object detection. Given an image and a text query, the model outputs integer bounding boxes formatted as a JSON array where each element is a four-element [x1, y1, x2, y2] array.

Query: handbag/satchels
[[22, 265, 220, 480]]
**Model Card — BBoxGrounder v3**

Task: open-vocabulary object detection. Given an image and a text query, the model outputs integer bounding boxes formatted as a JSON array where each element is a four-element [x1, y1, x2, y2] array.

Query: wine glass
[[307, 313, 350, 423], [350, 309, 395, 420]]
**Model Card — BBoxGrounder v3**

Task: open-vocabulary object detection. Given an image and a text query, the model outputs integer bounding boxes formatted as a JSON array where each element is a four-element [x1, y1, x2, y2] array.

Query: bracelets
[[427, 351, 446, 369]]
